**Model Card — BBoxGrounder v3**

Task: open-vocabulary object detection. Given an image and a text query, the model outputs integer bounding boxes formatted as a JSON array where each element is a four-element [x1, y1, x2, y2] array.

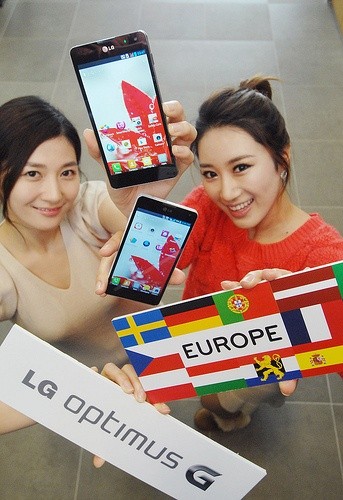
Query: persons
[[0, 96, 197, 438], [100, 72, 342, 429]]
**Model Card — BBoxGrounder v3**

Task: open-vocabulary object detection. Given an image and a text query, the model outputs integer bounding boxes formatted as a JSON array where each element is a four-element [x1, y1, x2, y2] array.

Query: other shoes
[[194, 407, 252, 432]]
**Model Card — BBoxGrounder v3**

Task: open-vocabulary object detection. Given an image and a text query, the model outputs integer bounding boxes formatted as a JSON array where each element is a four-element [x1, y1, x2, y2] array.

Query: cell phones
[[70, 28, 180, 190], [104, 194, 199, 305]]
